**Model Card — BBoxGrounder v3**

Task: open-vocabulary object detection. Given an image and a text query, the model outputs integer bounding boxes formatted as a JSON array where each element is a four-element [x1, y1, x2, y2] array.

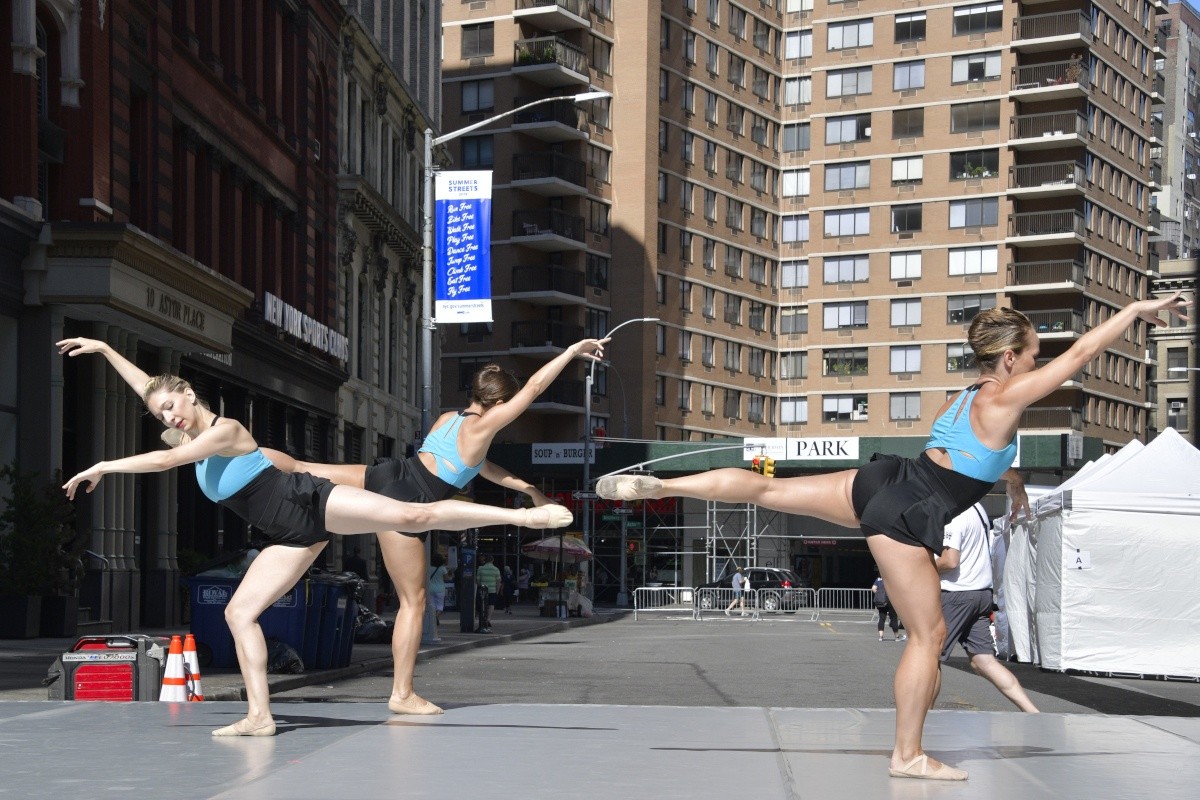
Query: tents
[[989, 425, 1200, 686]]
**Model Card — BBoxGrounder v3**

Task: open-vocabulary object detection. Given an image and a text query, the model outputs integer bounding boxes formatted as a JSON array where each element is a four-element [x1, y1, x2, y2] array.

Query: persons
[[593, 287, 1193, 781], [344, 547, 370, 584], [935, 501, 1045, 714], [427, 551, 530, 634], [725, 566, 749, 617], [54, 336, 572, 737], [159, 336, 613, 716], [871, 574, 906, 642]]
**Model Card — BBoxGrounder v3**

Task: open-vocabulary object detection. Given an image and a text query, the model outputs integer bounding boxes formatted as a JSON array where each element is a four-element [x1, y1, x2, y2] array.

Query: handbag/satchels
[[744, 580, 750, 592]]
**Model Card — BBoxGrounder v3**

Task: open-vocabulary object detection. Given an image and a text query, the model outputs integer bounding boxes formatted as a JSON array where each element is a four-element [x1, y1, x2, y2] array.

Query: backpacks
[[873, 580, 889, 608]]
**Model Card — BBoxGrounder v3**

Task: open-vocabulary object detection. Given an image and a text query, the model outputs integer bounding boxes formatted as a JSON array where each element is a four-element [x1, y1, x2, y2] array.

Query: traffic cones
[[159, 634, 203, 701]]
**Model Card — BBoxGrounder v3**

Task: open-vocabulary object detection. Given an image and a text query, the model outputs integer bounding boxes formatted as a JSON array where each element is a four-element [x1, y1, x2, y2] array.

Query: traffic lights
[[751, 457, 760, 474], [764, 458, 776, 478]]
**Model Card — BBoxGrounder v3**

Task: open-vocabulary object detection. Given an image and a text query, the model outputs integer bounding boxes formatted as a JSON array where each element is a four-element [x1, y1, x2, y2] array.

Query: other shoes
[[741, 612, 749, 617], [878, 636, 883, 641], [725, 609, 730, 616], [895, 634, 907, 642]]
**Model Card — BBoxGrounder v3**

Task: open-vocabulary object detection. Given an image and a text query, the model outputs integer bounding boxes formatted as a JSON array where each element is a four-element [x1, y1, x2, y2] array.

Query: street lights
[[424, 91, 614, 442], [580, 317, 662, 591]]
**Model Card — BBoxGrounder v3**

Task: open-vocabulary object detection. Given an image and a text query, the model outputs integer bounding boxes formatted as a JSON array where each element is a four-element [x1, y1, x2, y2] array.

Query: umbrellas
[[522, 534, 592, 586]]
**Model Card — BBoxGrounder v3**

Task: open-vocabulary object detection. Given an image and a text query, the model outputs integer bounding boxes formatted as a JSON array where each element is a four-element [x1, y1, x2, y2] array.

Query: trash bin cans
[[189, 564, 359, 671], [42, 629, 172, 703]]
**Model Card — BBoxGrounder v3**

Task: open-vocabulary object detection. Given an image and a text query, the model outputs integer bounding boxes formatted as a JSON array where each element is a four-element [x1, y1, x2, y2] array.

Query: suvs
[[693, 567, 804, 613]]
